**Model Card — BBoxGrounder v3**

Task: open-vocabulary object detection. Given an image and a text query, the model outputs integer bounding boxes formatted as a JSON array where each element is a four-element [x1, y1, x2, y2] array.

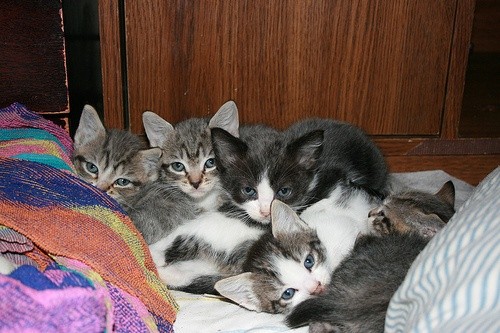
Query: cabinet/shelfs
[[97, 0, 500, 184]]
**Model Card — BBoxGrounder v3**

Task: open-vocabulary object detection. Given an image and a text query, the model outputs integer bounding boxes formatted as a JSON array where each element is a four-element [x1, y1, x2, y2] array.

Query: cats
[[73, 100, 456, 333]]
